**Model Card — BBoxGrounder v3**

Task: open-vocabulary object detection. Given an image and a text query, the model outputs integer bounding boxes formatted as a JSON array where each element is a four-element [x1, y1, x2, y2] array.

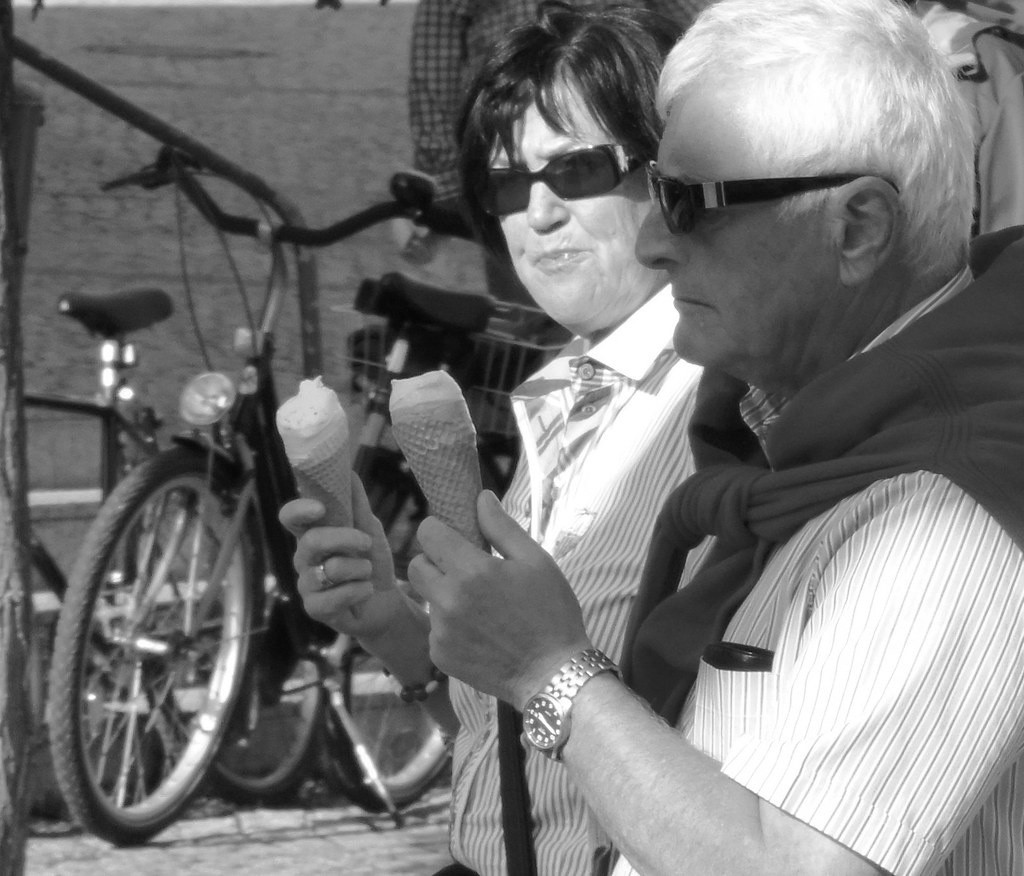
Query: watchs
[[521, 648, 625, 765]]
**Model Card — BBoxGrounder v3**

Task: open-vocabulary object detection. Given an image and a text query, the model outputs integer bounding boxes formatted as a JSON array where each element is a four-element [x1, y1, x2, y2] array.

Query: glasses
[[476, 143, 640, 216], [645, 159, 900, 237]]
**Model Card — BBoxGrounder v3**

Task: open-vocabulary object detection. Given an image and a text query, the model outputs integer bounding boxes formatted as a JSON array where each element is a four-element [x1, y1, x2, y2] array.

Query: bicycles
[[27, 137, 585, 848]]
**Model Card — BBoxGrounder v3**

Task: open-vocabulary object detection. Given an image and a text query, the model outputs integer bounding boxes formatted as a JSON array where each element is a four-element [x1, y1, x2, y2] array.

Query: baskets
[[335, 299, 574, 439]]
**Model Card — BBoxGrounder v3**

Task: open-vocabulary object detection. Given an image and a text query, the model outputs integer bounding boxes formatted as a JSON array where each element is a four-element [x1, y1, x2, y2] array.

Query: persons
[[410, 0, 1023, 876], [280, 0, 717, 876]]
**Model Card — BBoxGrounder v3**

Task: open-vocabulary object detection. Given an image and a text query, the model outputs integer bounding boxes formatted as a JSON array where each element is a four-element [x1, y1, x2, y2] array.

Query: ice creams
[[389, 370, 493, 555], [276, 375, 354, 530]]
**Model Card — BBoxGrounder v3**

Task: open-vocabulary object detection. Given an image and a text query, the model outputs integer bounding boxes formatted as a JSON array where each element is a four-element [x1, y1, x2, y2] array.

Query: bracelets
[[383, 669, 448, 704]]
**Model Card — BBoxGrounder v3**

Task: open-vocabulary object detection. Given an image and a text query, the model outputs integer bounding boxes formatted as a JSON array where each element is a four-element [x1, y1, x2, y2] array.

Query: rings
[[318, 562, 338, 588]]
